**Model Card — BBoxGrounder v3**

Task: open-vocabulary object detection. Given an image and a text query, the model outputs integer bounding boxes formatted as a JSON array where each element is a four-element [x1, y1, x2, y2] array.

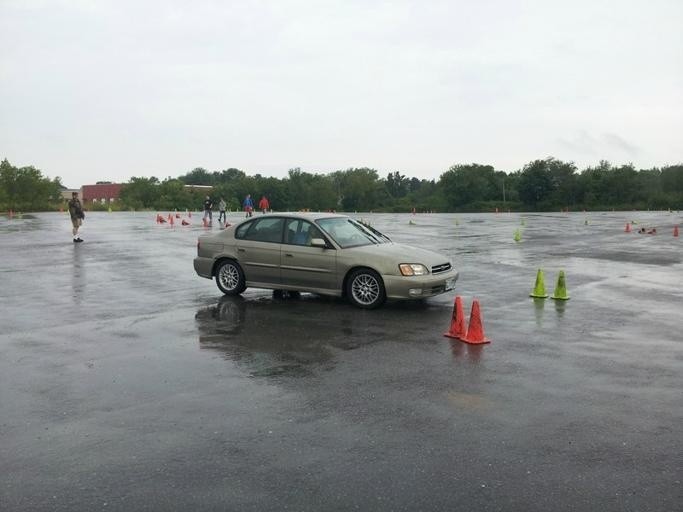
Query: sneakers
[[74, 238, 84, 243]]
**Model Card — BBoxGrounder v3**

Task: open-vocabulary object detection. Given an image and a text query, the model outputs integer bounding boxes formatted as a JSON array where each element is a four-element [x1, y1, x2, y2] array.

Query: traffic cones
[[512, 228, 523, 241], [583, 217, 590, 226], [551, 268, 571, 300], [623, 222, 632, 232], [154, 209, 233, 228], [526, 265, 552, 299], [298, 205, 422, 227], [519, 215, 528, 226], [635, 226, 658, 235], [672, 226, 680, 237], [9, 209, 15, 217], [458, 299, 493, 346], [453, 214, 461, 227], [108, 207, 113, 214], [436, 294, 467, 339]]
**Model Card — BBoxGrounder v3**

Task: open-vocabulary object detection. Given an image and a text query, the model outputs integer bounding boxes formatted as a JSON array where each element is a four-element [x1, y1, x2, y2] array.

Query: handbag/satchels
[[76, 207, 84, 219]]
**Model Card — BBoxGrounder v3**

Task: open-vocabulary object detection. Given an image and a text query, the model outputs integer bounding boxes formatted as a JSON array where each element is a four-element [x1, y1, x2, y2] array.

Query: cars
[[186, 208, 462, 312]]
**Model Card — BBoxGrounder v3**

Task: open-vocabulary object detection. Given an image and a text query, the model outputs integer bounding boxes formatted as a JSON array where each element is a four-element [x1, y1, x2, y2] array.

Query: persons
[[68, 191, 85, 242], [258, 195, 271, 215], [304, 226, 320, 246], [216, 196, 226, 222], [243, 194, 254, 218], [202, 195, 213, 222]]
